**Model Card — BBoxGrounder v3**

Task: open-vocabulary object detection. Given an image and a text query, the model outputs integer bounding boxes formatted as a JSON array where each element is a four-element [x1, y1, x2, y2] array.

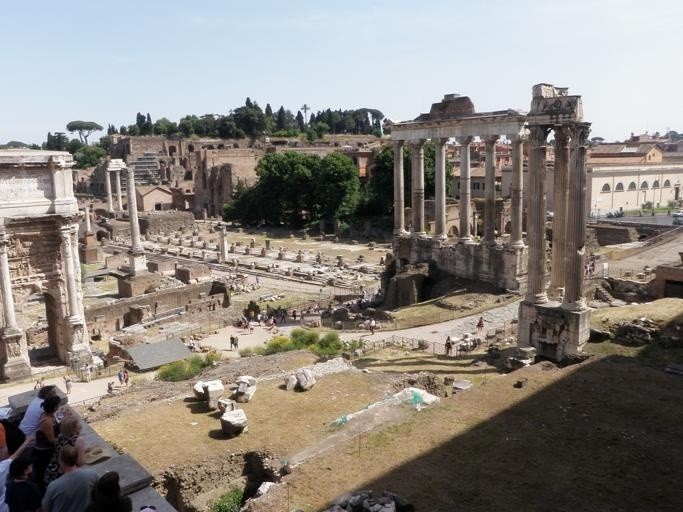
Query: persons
[[33, 381, 40, 390], [239, 309, 296, 335], [123, 369, 129, 386], [591, 260, 595, 274], [65, 377, 72, 394], [84, 362, 91, 383], [230, 334, 235, 349], [39, 377, 44, 387], [118, 369, 123, 385], [585, 261, 589, 275], [189, 336, 194, 349], [443, 335, 454, 355], [234, 336, 238, 348], [369, 317, 375, 336], [0, 384, 157, 512], [476, 316, 484, 330]]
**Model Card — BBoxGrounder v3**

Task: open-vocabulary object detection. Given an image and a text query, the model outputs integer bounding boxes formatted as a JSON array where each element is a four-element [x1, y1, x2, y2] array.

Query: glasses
[[140, 506, 156, 511]]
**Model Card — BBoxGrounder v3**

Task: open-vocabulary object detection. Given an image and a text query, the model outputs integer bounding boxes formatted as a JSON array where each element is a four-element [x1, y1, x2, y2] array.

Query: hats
[[140, 508, 156, 512], [84, 445, 111, 464]]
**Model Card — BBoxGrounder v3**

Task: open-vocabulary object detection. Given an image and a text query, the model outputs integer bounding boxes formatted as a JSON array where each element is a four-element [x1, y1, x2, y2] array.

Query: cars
[[545, 210, 553, 218], [673, 210, 683, 226]]
